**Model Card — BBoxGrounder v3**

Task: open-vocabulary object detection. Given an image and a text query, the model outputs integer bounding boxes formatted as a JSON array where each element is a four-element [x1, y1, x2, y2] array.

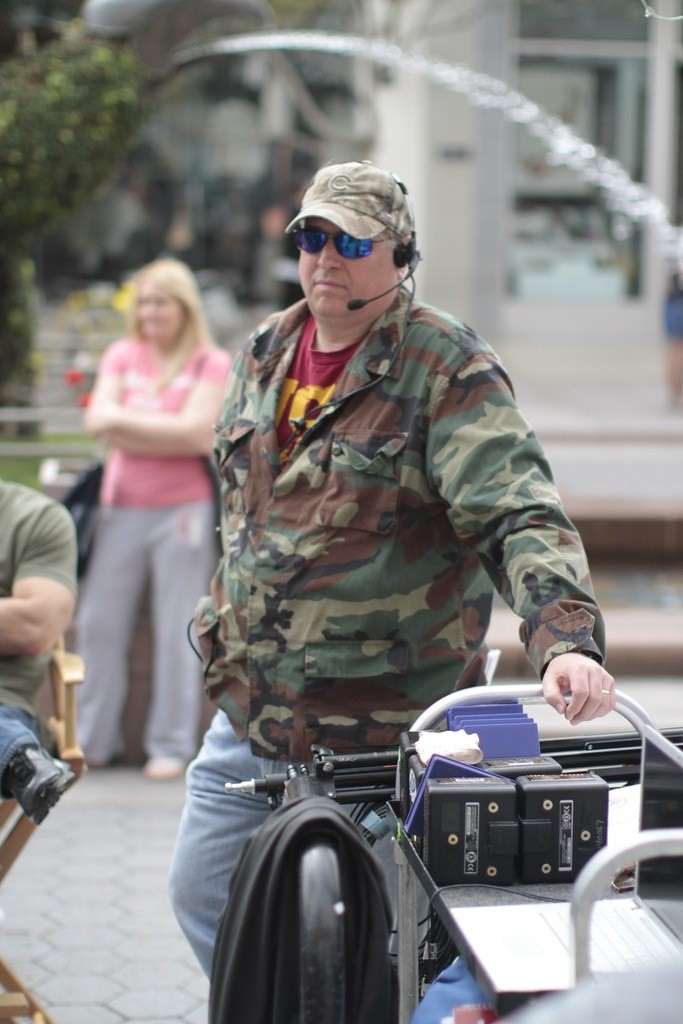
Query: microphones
[[345, 260, 418, 311]]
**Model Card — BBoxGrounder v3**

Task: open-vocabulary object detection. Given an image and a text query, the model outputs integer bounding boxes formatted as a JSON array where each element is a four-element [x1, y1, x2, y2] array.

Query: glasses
[[293, 228, 390, 260]]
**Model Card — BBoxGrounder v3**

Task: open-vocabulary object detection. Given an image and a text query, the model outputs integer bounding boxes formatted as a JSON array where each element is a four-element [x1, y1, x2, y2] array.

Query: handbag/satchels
[[61, 463, 105, 580]]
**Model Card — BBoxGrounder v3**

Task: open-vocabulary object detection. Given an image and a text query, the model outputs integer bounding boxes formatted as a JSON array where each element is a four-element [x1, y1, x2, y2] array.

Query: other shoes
[[143, 756, 185, 781]]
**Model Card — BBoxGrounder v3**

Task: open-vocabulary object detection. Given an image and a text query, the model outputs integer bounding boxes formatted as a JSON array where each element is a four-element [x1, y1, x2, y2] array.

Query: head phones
[[283, 175, 421, 269]]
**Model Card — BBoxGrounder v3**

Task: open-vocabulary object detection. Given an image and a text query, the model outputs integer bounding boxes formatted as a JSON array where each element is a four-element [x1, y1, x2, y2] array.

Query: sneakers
[[4, 745, 74, 824]]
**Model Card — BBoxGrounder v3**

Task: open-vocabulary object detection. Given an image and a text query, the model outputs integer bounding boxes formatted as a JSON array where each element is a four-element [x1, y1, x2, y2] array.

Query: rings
[[602, 689, 610, 695]]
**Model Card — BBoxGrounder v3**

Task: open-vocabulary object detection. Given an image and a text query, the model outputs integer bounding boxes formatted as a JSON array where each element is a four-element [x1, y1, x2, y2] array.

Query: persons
[[73, 256, 233, 777], [0, 477, 77, 822], [168, 161, 618, 979]]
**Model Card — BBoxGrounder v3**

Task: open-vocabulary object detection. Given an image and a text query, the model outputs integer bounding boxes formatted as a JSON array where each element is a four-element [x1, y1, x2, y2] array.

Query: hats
[[286, 160, 414, 240]]
